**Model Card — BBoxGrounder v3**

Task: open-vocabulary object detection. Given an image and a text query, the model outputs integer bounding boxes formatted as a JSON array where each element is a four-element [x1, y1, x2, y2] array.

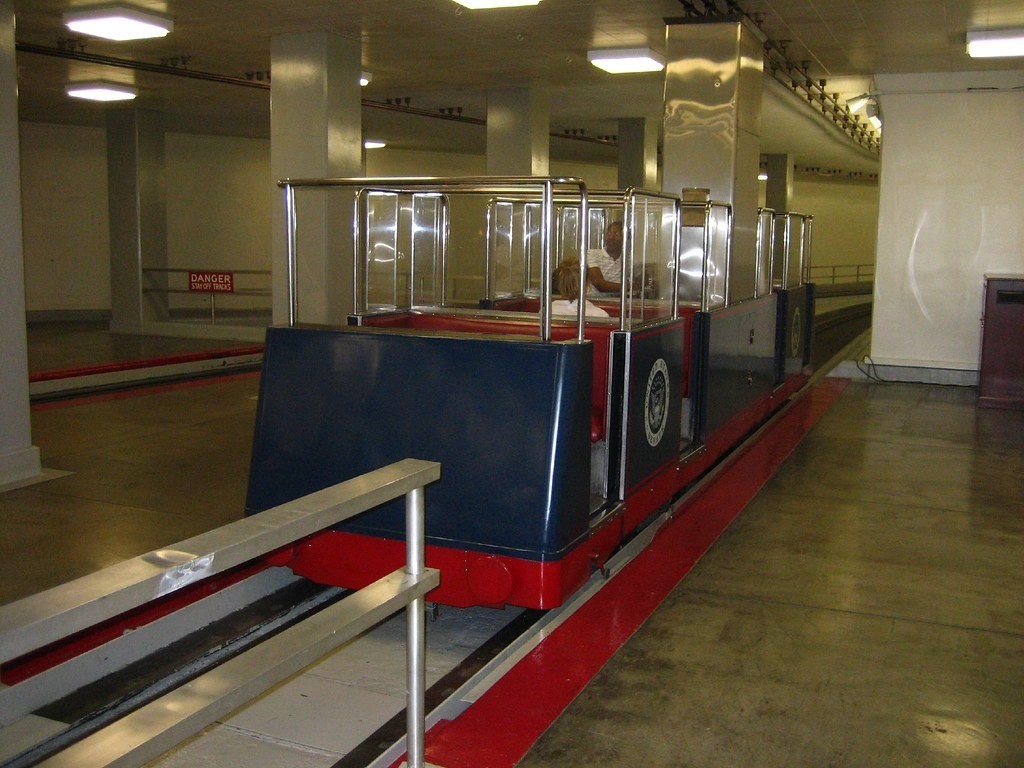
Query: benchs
[[363, 294, 702, 442]]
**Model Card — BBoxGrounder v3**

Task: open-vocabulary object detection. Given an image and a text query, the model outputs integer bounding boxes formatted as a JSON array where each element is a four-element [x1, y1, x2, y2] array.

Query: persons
[[586, 221, 642, 298], [538, 254, 609, 318]]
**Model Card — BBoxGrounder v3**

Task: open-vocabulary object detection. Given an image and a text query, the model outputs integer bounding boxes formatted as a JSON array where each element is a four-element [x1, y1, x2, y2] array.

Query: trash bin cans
[[975, 273, 1024, 412]]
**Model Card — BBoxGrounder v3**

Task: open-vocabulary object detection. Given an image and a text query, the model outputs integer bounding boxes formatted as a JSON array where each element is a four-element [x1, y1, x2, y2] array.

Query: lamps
[[360, 71, 372, 86], [966, 29, 1024, 58], [63, 8, 174, 42], [365, 139, 387, 148], [846, 93, 871, 113], [587, 48, 666, 73], [66, 83, 139, 101]]
